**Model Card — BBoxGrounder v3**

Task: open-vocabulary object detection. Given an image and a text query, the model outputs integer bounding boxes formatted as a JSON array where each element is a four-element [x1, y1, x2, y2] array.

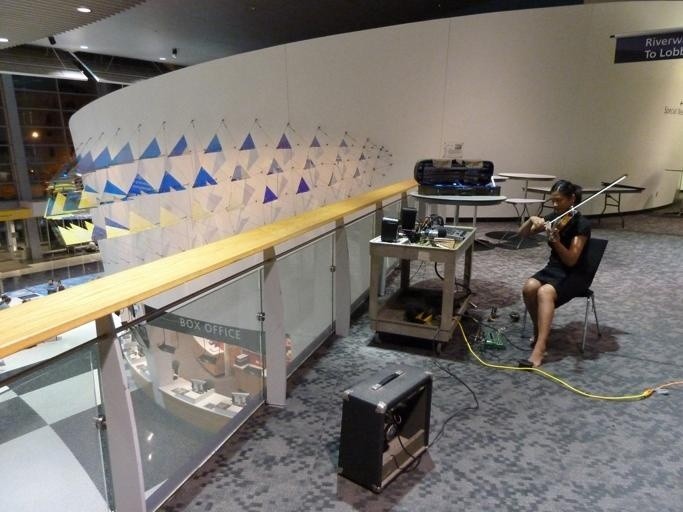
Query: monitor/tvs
[[232, 393, 249, 407], [190, 379, 207, 393]]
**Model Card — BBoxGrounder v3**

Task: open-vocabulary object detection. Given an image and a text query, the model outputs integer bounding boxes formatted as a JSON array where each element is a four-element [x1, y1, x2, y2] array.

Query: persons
[[512, 179, 593, 370], [0, 298, 12, 310], [284, 346, 294, 363], [0, 295, 8, 305], [44, 279, 58, 295], [284, 332, 294, 348], [56, 280, 65, 291]]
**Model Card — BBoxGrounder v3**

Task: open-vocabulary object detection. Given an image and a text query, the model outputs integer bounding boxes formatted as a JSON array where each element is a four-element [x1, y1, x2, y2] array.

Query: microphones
[[412, 229, 446, 237]]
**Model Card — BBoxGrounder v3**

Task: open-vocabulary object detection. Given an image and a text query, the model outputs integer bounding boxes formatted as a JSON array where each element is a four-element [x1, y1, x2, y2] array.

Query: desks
[[415, 172, 556, 250], [522, 181, 645, 228]]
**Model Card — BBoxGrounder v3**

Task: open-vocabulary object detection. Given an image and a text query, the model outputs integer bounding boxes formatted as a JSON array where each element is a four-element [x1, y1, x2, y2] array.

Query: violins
[[549, 208, 578, 241]]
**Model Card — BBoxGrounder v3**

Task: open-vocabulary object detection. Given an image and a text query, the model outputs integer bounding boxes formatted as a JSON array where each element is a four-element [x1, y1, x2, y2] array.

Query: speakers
[[401, 207, 417, 229], [336, 362, 432, 491], [381, 217, 399, 243]]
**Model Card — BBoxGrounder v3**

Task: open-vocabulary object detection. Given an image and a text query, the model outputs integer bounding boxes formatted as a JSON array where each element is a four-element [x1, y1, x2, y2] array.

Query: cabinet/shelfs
[[368, 221, 478, 342]]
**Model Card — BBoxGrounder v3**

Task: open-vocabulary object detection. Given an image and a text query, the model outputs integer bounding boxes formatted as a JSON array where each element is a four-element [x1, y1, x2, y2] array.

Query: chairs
[[520, 237, 609, 354]]
